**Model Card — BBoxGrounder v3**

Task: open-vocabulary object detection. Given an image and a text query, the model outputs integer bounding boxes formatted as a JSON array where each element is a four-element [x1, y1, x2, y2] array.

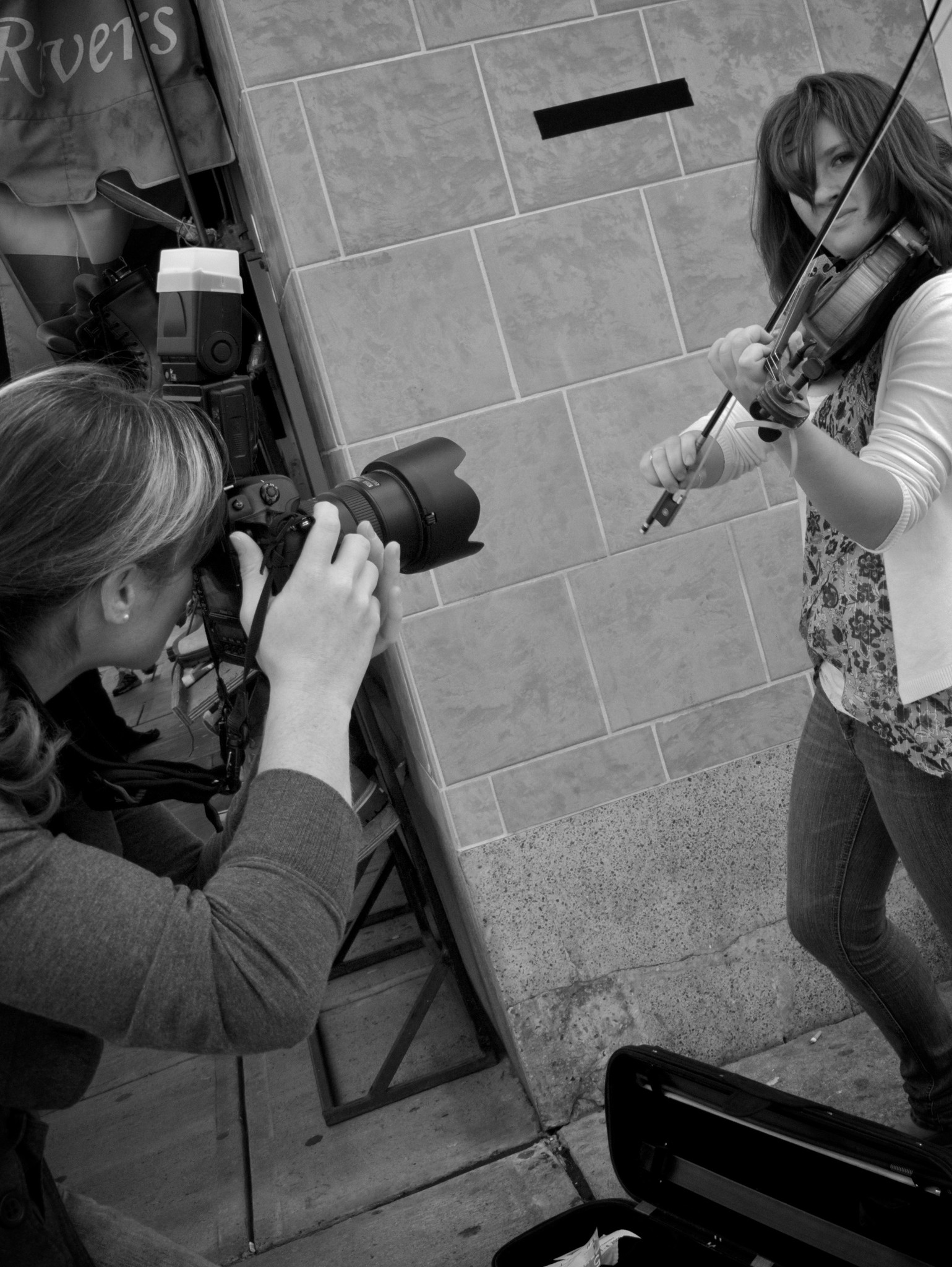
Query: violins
[[749, 212, 935, 445]]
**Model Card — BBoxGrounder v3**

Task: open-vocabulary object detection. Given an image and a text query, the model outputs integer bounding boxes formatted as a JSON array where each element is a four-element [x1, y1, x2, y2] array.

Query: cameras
[[219, 436, 483, 614]]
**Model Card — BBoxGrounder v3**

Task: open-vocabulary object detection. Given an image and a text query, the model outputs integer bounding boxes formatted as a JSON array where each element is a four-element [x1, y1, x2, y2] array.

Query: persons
[[111, 663, 159, 697], [633, 72, 951, 1152], [1, 364, 406, 1267]]
